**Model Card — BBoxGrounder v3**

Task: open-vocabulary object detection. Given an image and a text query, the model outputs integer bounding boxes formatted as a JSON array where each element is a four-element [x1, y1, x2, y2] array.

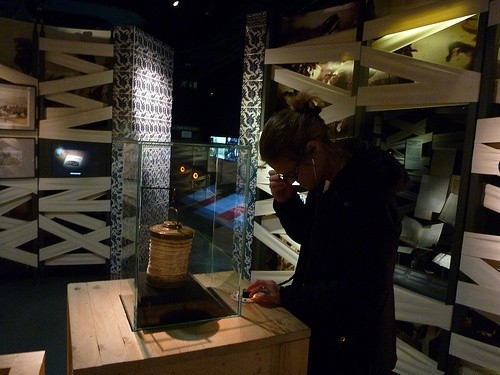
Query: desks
[[66, 280, 312, 375]]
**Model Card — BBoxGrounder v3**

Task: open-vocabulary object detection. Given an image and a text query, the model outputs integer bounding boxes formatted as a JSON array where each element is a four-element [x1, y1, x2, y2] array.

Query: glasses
[[283, 158, 302, 182]]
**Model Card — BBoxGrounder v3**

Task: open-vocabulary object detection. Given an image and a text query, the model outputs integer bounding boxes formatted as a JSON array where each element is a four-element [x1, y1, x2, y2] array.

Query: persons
[[247, 87, 404, 375]]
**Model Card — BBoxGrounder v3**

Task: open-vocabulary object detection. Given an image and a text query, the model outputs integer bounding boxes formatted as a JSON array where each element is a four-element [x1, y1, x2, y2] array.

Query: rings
[[260, 284, 268, 293]]
